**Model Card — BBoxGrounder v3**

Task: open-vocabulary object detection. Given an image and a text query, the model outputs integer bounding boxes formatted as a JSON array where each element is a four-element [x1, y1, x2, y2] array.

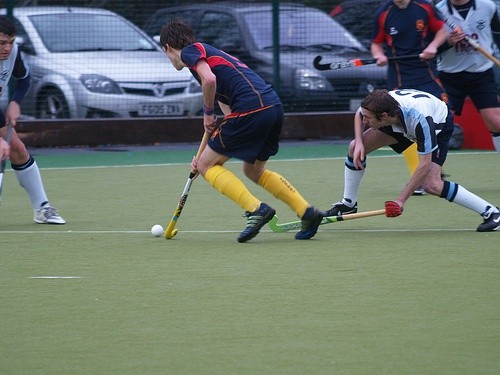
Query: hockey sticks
[[313, 53, 422, 71], [0, 120, 12, 197], [165, 113, 218, 239], [268, 206, 405, 233], [426, 0, 500, 68]]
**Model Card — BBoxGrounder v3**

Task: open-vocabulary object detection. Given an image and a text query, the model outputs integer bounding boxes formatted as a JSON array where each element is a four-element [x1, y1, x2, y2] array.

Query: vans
[[145, 2, 390, 114]]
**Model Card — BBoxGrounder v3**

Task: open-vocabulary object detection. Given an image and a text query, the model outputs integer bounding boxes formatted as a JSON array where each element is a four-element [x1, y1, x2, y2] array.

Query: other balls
[[151, 224, 164, 237]]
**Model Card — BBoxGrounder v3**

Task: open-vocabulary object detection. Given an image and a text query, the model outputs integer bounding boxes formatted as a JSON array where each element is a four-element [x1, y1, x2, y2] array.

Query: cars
[[329, 0, 500, 95], [0, 7, 206, 118]]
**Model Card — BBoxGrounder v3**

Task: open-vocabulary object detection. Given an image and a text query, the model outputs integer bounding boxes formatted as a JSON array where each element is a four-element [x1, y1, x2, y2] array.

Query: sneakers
[[33, 206, 67, 225], [295, 206, 324, 240], [237, 203, 276, 243], [476, 205, 500, 232], [411, 185, 426, 196], [324, 198, 357, 217]]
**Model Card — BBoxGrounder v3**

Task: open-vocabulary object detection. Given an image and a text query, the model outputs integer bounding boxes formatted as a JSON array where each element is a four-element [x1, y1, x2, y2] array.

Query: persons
[[324, 89, 500, 232], [0, 15, 66, 224], [160, 21, 324, 243], [371, 0, 500, 154]]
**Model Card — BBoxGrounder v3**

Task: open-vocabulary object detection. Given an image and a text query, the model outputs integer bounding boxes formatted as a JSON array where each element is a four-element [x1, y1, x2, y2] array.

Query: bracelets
[[202, 103, 214, 115]]
